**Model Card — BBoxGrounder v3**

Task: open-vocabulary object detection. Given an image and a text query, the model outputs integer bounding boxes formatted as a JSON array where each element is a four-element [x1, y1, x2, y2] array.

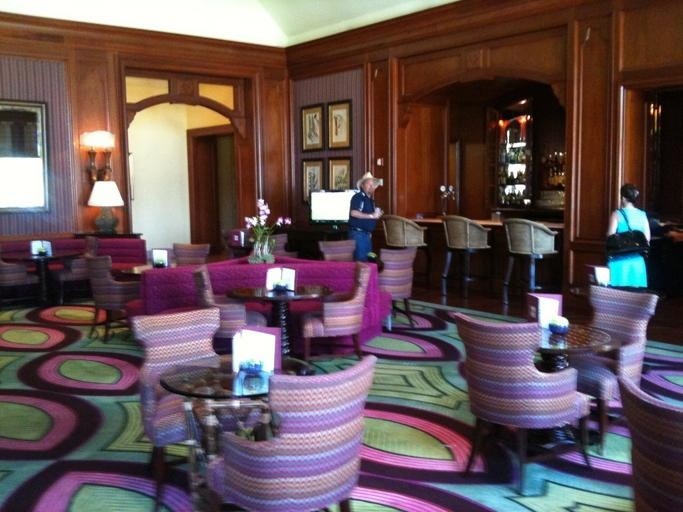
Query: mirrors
[[0, 99, 50, 215]]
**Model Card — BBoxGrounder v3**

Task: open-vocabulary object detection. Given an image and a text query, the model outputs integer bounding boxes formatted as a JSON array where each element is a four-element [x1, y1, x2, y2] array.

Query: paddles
[[1, 281, 683, 512]]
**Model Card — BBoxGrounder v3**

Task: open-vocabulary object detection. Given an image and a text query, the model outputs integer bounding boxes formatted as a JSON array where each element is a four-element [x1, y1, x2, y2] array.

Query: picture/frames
[[4, 250, 82, 306], [299, 102, 324, 154], [301, 157, 325, 206], [326, 98, 353, 150], [327, 155, 354, 193], [74, 232, 143, 239]]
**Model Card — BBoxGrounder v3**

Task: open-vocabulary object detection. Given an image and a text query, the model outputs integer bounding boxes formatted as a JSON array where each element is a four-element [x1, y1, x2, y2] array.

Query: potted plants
[[301, 157, 325, 206], [326, 98, 353, 150], [327, 155, 354, 193], [299, 102, 324, 154]]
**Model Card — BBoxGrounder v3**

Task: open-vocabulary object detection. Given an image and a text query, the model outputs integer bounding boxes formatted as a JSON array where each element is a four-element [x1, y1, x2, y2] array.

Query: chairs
[[503, 218, 559, 289], [83, 212, 415, 369], [382, 215, 432, 279], [59, 235, 99, 304], [618, 376, 681, 512], [1, 259, 39, 306], [132, 308, 272, 511], [565, 285, 658, 456], [452, 312, 595, 496], [206, 355, 377, 512], [443, 215, 493, 278]]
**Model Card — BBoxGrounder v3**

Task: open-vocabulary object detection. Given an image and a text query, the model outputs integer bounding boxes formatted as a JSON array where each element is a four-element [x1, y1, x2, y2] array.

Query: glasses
[[86, 181, 126, 235], [81, 130, 115, 185]]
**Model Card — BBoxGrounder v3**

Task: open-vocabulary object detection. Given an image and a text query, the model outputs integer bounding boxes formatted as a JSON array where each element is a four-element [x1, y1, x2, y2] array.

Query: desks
[[407, 217, 565, 283], [74, 232, 143, 239], [535, 324, 612, 450], [4, 250, 82, 306], [159, 355, 315, 512]]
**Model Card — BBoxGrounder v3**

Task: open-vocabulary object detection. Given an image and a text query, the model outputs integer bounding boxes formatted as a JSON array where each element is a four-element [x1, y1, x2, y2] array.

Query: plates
[[500, 155, 528, 188]]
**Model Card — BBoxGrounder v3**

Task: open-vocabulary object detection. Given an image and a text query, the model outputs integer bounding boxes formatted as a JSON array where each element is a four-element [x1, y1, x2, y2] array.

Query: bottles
[[356, 172, 382, 192]]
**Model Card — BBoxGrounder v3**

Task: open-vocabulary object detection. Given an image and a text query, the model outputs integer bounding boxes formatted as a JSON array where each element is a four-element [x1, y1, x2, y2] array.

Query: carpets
[[1, 281, 683, 512]]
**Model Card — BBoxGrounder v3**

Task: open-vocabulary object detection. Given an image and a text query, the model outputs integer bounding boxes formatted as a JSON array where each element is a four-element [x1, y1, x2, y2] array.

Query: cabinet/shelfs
[[541, 150, 567, 176], [500, 155, 528, 188], [497, 145, 529, 205]]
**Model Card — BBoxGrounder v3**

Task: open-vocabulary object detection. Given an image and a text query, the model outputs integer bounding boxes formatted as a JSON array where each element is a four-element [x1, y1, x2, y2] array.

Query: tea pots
[[0, 99, 50, 215]]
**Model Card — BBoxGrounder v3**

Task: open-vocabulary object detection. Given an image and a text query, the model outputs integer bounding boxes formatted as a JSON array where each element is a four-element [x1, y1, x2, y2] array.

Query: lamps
[[86, 181, 126, 235], [59, 235, 99, 304], [81, 130, 115, 185], [1, 259, 39, 306]]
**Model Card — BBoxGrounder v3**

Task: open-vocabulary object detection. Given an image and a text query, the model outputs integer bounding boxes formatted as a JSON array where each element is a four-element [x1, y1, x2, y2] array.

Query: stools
[[348, 226, 367, 232]]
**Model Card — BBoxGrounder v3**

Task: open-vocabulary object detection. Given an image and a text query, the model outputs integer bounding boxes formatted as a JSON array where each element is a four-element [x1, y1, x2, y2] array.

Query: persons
[[607, 183, 651, 290], [348, 171, 384, 261]]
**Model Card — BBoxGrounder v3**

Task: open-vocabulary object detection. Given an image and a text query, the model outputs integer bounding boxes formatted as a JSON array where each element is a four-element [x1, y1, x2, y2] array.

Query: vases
[[240, 198, 293, 240]]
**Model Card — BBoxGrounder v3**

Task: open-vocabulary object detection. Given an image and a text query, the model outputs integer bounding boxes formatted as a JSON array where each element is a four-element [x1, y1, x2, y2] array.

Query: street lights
[[605, 208, 649, 258]]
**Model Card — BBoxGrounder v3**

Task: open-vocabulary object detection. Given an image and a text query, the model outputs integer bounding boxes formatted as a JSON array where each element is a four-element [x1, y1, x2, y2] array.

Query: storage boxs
[[0, 239, 145, 279]]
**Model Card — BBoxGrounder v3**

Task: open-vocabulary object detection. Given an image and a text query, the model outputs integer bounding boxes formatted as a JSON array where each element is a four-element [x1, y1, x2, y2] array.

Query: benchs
[[0, 239, 145, 279]]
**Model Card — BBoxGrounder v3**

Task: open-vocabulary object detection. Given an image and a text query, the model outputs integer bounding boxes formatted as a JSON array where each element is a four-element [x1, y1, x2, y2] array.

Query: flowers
[[240, 198, 293, 240]]
